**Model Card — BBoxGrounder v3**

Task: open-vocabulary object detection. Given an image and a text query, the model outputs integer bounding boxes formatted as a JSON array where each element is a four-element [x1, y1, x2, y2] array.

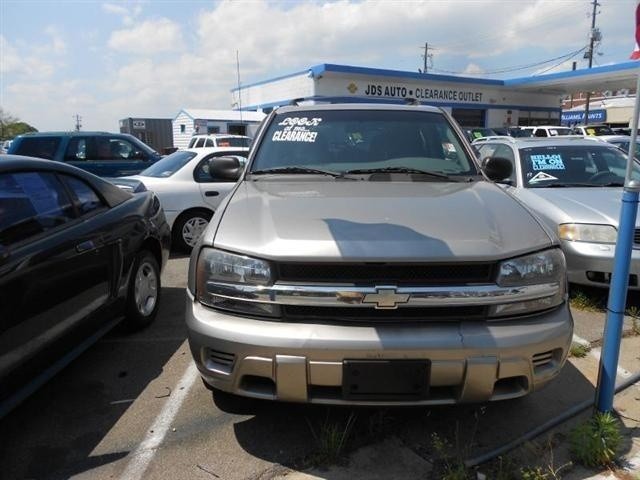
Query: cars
[[1, 150, 177, 438], [471, 135, 640, 300], [115, 147, 249, 257], [459, 124, 640, 165]]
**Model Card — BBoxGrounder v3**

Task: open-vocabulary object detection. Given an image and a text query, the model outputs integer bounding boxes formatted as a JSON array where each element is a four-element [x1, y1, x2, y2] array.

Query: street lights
[[582, 48, 604, 125]]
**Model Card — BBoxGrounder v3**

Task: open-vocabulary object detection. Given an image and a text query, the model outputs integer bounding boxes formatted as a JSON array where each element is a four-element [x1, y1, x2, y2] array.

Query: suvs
[[7, 129, 167, 177], [188, 133, 253, 148], [178, 93, 579, 423]]
